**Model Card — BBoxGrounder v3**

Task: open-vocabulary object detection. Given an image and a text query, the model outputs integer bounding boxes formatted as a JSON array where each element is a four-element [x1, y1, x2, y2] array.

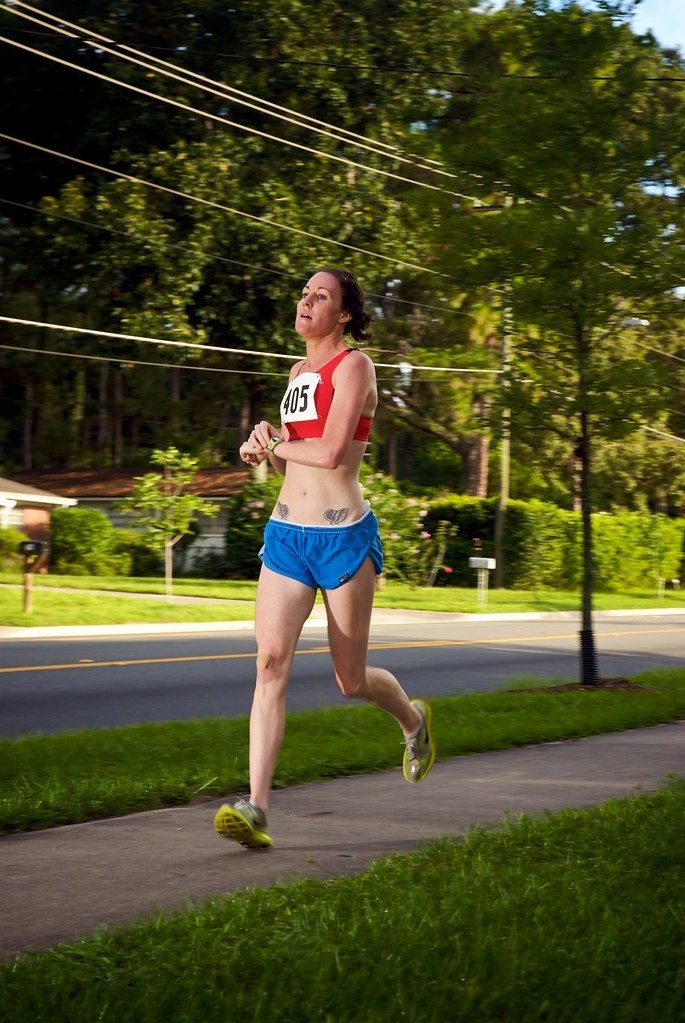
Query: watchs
[[264, 436, 282, 457]]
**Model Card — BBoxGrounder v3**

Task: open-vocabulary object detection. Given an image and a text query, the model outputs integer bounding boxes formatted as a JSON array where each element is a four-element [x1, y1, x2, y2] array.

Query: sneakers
[[400, 700, 435, 784], [214, 796, 273, 850]]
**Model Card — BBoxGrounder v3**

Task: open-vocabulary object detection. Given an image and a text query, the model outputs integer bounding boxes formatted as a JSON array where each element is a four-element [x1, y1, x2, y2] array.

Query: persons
[[209, 264, 434, 854]]
